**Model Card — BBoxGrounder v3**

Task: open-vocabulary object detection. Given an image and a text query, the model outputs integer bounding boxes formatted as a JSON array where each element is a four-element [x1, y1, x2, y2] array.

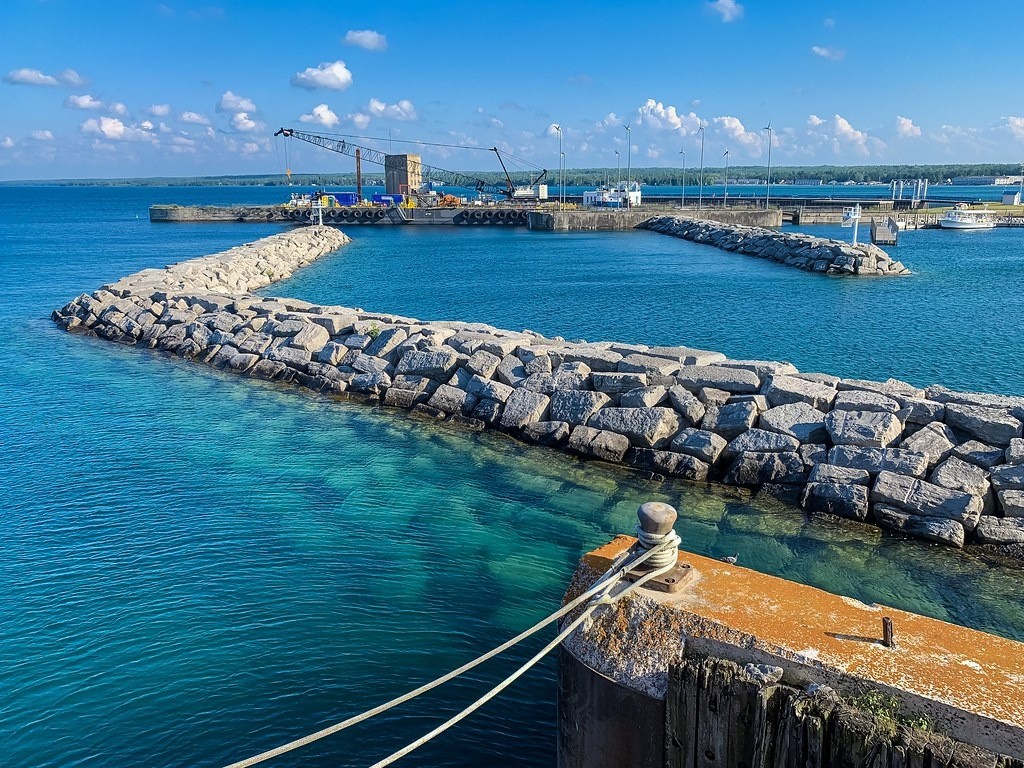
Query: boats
[[938, 202, 1002, 227]]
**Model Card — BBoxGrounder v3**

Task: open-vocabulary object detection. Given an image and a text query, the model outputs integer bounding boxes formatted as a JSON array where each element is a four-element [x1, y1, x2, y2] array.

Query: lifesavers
[[364, 210, 374, 219], [329, 209, 338, 219], [305, 209, 312, 218], [281, 209, 290, 217], [293, 209, 301, 218], [510, 210, 519, 220], [462, 210, 470, 219], [521, 211, 529, 220], [352, 210, 362, 220], [322, 209, 326, 219], [474, 210, 483, 220], [377, 209, 386, 218], [497, 210, 507, 220], [485, 211, 494, 221], [341, 210, 350, 218]]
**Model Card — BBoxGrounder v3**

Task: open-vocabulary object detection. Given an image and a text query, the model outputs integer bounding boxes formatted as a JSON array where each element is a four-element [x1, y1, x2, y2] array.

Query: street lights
[[762, 119, 771, 210], [696, 120, 705, 209], [622, 122, 631, 211], [553, 123, 565, 211], [615, 150, 621, 209], [721, 147, 729, 209], [677, 145, 686, 208]]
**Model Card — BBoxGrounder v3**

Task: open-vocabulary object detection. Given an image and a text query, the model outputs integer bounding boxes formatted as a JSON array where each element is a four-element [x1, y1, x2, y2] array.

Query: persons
[[291, 192, 298, 207]]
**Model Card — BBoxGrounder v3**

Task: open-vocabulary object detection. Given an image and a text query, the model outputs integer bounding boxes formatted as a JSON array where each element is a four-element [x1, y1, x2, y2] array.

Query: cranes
[[274, 127, 548, 199]]
